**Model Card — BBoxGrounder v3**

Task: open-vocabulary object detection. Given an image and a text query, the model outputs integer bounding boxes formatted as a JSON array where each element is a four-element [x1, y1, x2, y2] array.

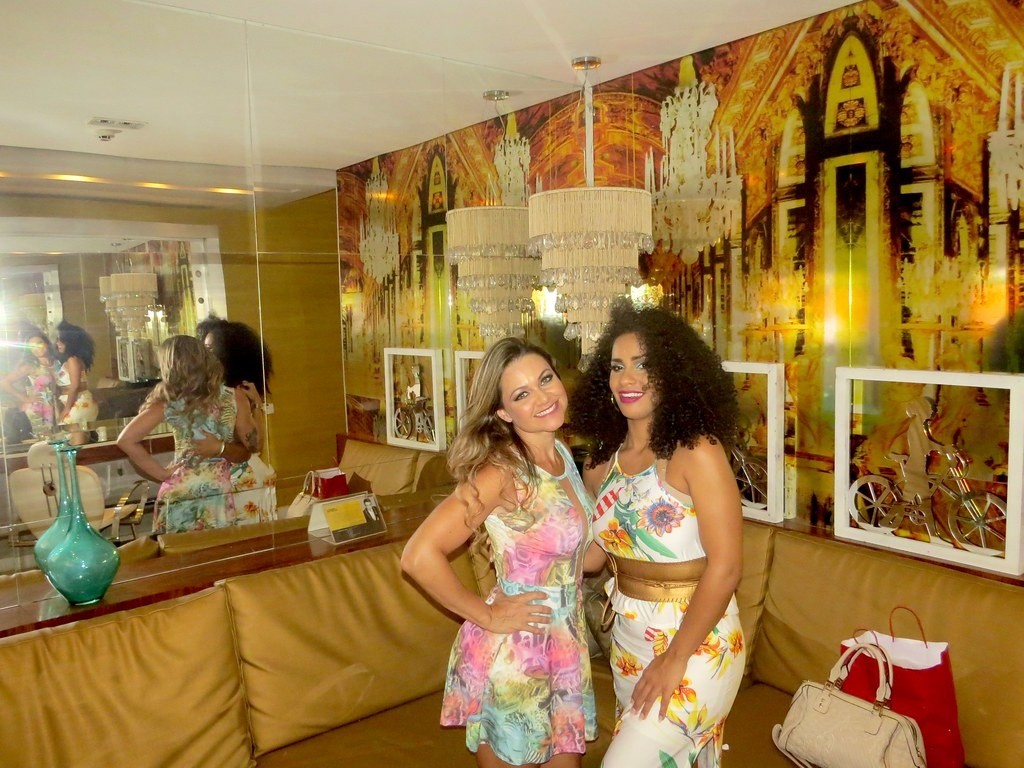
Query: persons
[[401, 337, 608, 767], [574, 304, 746, 767], [116, 316, 281, 533], [362, 498, 380, 523], [1, 319, 100, 434]]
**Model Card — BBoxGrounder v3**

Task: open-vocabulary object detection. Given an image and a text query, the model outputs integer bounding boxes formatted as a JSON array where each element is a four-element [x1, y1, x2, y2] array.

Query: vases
[[33, 438, 128, 607]]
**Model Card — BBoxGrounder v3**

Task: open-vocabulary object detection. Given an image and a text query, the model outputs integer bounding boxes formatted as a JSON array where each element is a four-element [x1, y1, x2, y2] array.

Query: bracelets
[[219, 440, 225, 459]]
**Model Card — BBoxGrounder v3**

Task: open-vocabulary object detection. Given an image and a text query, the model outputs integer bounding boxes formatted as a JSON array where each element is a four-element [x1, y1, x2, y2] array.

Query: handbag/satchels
[[840, 606, 964, 768], [771, 643, 928, 768], [284, 471, 321, 518], [309, 466, 349, 498]]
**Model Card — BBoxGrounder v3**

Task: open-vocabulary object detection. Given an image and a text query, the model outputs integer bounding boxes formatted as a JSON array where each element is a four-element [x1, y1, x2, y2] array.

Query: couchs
[[33, 521, 980, 768]]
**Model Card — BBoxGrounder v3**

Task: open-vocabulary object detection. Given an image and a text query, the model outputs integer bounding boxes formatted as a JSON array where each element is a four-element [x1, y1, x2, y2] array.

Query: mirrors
[[2, 217, 230, 455]]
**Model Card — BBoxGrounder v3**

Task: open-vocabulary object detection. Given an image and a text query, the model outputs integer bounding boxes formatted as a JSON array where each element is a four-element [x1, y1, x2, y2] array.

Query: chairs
[[9, 440, 142, 544]]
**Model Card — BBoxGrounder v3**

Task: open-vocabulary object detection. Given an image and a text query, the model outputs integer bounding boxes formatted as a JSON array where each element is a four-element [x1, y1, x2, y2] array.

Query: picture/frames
[[455, 352, 493, 433], [837, 365, 1024, 574], [688, 361, 785, 521], [385, 347, 444, 448]]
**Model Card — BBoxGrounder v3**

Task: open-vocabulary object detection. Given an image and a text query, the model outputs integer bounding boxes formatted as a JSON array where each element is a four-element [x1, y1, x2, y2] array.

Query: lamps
[[112, 262, 159, 342], [446, 97, 528, 340], [529, 54, 654, 345], [98, 270, 115, 330]]
